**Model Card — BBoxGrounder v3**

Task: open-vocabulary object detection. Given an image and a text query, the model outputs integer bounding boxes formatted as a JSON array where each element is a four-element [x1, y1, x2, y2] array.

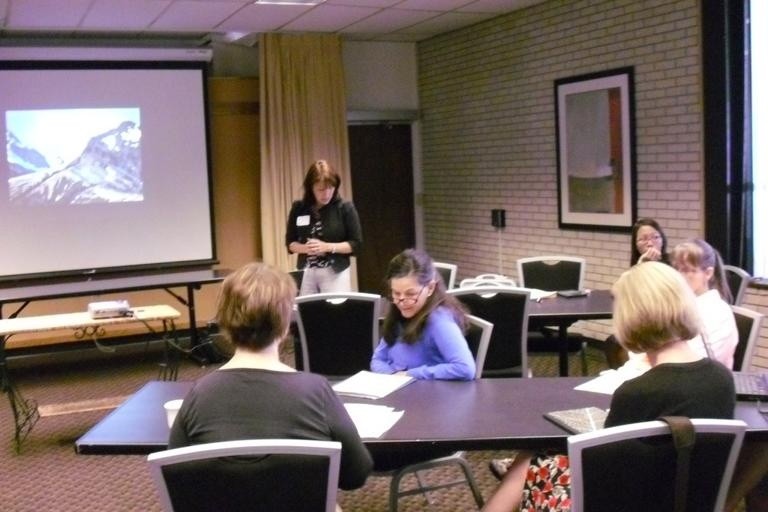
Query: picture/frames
[[553, 65, 638, 236]]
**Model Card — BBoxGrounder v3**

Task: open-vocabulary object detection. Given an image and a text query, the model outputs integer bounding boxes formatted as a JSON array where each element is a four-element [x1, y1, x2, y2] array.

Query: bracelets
[[332, 244, 338, 253]]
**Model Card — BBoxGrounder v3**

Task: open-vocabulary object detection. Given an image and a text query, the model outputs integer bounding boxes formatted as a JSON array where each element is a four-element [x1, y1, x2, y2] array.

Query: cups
[[164, 398, 184, 429]]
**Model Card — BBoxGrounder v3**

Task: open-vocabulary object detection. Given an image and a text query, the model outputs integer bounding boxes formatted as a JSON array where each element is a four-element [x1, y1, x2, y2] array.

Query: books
[[728, 369, 762, 406], [543, 406, 610, 435]]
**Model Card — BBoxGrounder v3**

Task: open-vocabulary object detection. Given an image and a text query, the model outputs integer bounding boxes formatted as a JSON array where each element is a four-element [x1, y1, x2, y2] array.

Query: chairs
[[293, 291, 383, 381], [430, 261, 458, 292], [443, 284, 532, 380], [722, 264, 752, 307], [516, 255, 587, 293], [566, 417, 748, 511], [148, 436, 344, 512], [366, 312, 495, 512]]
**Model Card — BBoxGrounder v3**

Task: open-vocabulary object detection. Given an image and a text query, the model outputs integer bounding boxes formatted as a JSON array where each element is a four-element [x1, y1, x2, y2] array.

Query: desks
[[522, 288, 617, 378], [71, 370, 768, 458], [0, 267, 235, 392], [0, 303, 182, 394]]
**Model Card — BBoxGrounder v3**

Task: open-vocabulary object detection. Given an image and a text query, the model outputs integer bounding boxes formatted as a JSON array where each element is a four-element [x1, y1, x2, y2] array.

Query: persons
[[169, 263, 374, 512], [479, 260, 736, 512], [370, 248, 477, 380], [629, 217, 672, 265], [488, 239, 738, 482], [284, 159, 364, 297]]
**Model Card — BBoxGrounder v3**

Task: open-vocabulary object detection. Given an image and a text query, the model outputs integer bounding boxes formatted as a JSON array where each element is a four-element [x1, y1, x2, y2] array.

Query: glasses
[[675, 265, 703, 278], [637, 234, 661, 242], [390, 285, 424, 305]]
